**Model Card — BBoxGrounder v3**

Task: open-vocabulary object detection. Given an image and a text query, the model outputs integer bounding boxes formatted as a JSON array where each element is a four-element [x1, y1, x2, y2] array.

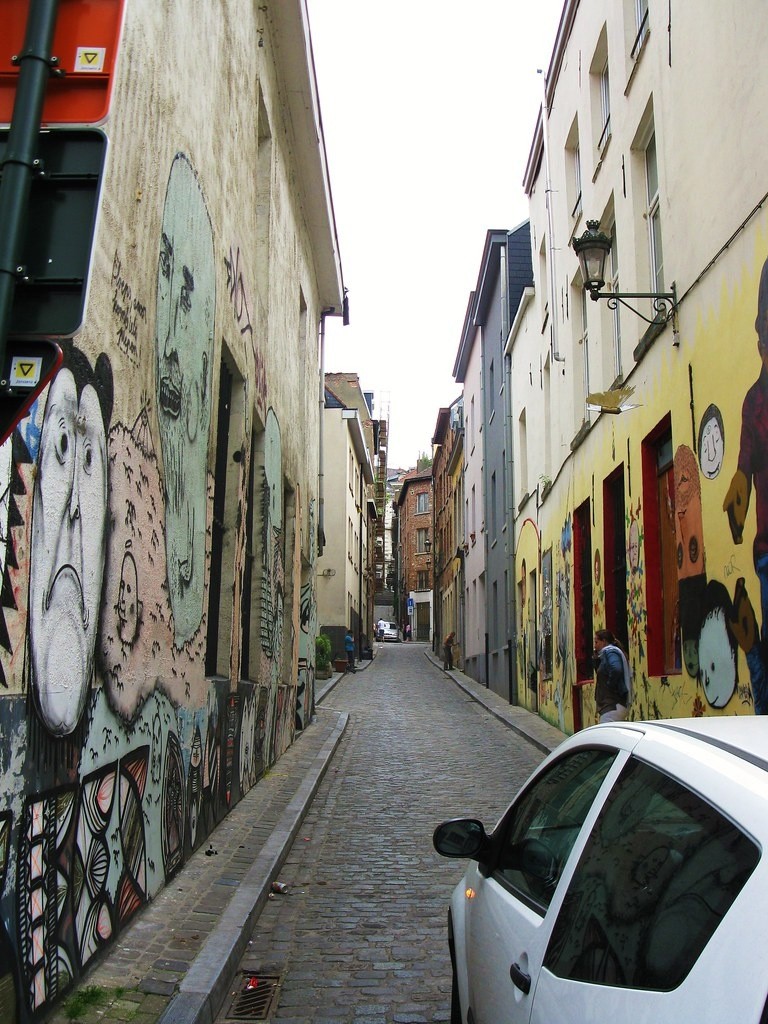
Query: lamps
[[424, 539, 440, 555], [572, 219, 680, 349]]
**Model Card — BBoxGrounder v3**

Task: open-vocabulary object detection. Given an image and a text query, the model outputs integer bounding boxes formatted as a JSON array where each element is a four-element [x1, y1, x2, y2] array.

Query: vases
[[570, 420, 590, 450], [518, 493, 529, 510]]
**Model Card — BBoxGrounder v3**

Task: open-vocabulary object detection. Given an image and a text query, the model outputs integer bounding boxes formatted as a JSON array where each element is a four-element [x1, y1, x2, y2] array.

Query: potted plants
[[314, 634, 333, 680], [539, 473, 552, 500], [334, 655, 349, 672]]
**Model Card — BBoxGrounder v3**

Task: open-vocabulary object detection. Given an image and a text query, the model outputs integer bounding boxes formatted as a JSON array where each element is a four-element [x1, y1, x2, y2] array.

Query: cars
[[378, 622, 399, 642], [431, 713, 768, 1024]]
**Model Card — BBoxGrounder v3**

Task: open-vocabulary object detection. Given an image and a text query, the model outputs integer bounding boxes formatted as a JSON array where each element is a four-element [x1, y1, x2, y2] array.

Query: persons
[[443, 631, 459, 671], [345, 630, 358, 673], [592, 629, 633, 726], [373, 618, 410, 643]]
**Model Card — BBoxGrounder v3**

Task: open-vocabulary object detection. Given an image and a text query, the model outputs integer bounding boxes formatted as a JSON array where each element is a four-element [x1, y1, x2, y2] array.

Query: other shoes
[[449, 667, 455, 670], [444, 668, 449, 671]]
